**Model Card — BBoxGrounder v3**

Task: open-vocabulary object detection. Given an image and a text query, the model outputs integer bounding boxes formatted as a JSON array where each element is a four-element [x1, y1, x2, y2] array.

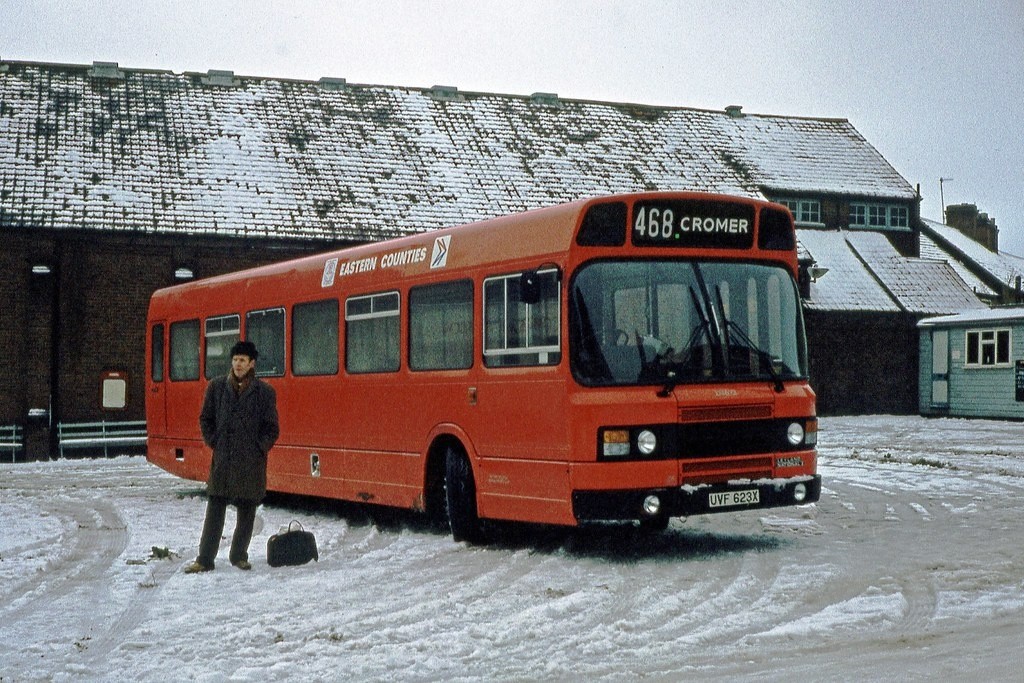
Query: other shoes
[[232, 559, 251, 570], [185, 562, 214, 573]]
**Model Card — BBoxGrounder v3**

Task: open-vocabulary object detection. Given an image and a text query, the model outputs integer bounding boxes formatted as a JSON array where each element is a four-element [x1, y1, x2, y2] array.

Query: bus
[[145, 191, 822, 545]]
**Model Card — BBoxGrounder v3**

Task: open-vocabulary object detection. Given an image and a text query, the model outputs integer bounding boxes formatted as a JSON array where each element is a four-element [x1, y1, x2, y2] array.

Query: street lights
[[940, 177, 953, 226], [32, 242, 65, 462]]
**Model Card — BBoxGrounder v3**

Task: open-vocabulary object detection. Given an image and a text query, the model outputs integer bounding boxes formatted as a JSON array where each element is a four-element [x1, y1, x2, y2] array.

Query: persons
[[185, 342, 279, 572]]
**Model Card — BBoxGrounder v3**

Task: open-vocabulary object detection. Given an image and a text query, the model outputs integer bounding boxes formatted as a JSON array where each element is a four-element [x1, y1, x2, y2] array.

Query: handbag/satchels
[[267, 520, 318, 568]]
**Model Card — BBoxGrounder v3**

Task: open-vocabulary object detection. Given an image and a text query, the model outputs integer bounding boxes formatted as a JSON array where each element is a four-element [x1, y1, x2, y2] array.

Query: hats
[[231, 342, 257, 360]]
